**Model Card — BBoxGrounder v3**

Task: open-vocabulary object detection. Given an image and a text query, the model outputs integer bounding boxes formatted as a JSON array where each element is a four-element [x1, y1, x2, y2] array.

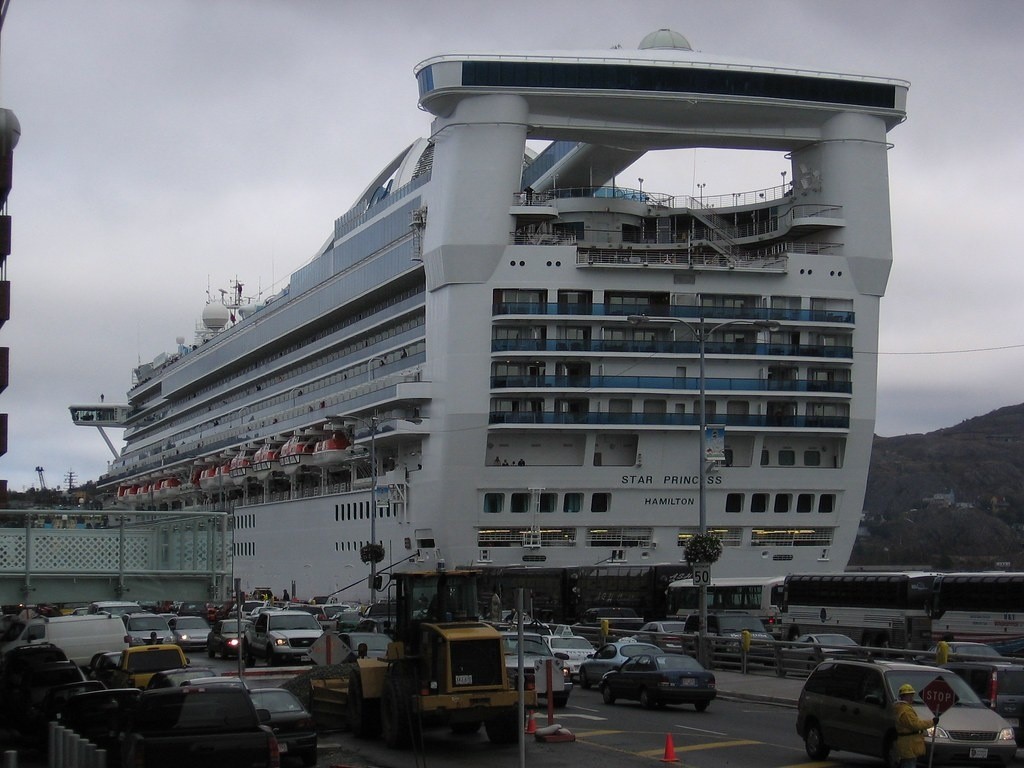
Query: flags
[[230, 314, 237, 324]]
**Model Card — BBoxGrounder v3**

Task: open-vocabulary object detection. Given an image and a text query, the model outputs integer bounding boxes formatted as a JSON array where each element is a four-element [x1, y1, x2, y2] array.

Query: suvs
[[794, 656, 1017, 768]]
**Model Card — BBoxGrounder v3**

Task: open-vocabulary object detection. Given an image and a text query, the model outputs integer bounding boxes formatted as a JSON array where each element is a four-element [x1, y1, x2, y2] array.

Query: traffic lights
[[374, 576, 382, 590], [227, 638, 240, 646], [525, 673, 537, 691]]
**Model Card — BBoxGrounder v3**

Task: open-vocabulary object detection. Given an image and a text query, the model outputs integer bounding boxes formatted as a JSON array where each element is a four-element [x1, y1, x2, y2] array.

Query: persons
[[891, 684, 939, 768], [254, 588, 290, 606], [100, 394, 104, 403], [524, 185, 535, 206], [147, 631, 163, 645], [420, 594, 428, 609], [113, 338, 408, 471], [936, 633, 953, 666]]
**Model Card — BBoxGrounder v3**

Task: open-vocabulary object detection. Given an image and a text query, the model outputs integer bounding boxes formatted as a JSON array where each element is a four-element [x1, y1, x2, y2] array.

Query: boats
[[314, 433, 351, 452], [279, 434, 319, 456], [228, 453, 251, 471], [253, 443, 281, 461], [116, 465, 228, 499]]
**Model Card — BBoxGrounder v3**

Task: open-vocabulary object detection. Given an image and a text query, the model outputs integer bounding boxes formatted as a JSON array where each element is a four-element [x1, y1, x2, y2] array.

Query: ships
[[69, 26, 911, 605]]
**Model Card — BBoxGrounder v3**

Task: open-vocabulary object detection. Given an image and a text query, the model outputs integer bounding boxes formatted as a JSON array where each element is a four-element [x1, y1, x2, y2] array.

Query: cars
[[0, 562, 1023, 768], [577, 642, 666, 689], [599, 653, 720, 710]]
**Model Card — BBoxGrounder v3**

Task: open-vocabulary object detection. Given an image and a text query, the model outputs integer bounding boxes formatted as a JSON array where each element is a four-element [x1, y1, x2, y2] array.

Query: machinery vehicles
[[306, 558, 539, 751]]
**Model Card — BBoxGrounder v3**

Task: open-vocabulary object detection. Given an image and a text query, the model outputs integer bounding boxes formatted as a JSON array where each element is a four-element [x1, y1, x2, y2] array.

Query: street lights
[[322, 413, 423, 604], [626, 313, 784, 666]]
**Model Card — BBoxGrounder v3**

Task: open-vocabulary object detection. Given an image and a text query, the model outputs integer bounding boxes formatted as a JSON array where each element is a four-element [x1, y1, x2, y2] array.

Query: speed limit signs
[[692, 562, 712, 585]]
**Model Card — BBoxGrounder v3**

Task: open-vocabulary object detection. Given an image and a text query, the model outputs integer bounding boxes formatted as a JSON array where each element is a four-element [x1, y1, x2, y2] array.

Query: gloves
[[932, 717, 939, 726]]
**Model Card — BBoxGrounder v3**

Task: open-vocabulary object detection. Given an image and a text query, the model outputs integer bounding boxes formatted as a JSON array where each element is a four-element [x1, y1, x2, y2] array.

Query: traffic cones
[[525, 710, 538, 734], [659, 733, 681, 762]]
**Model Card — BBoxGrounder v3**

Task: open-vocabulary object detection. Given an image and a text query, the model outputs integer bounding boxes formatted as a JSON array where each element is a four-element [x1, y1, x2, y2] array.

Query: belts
[[898, 730, 923, 737]]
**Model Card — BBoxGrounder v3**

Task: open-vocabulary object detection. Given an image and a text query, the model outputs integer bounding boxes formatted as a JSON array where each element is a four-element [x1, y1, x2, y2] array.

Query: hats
[[150, 632, 156, 637]]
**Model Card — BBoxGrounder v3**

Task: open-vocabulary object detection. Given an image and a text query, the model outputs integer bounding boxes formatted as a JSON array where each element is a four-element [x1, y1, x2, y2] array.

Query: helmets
[[898, 684, 915, 697]]
